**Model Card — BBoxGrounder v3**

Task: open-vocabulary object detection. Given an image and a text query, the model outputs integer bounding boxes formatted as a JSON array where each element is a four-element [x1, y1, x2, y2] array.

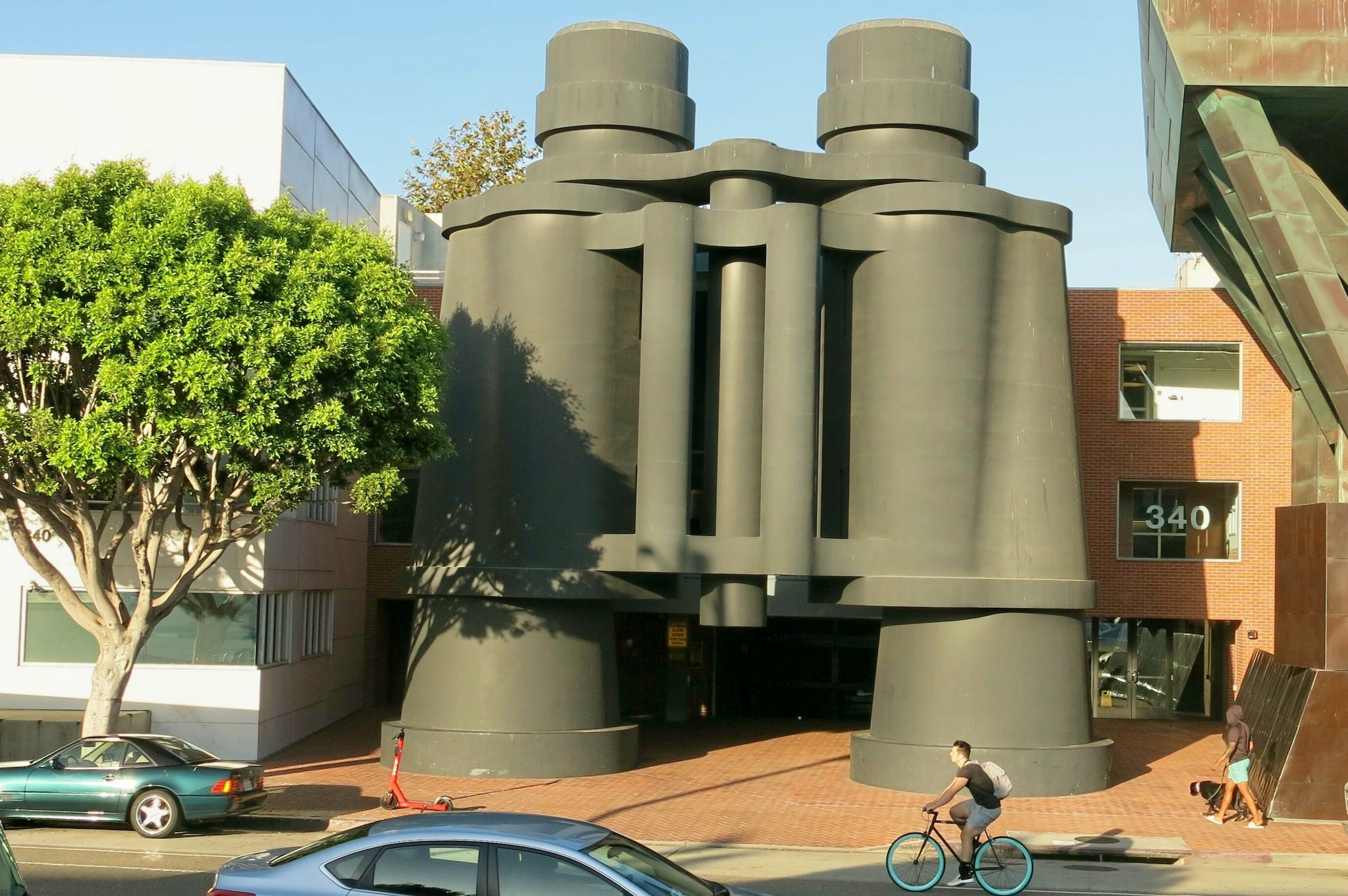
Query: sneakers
[[946, 872, 974, 886], [972, 839, 984, 854]]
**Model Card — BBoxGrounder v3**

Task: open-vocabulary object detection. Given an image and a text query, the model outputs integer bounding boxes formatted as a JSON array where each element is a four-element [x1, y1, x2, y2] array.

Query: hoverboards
[[380, 727, 453, 813]]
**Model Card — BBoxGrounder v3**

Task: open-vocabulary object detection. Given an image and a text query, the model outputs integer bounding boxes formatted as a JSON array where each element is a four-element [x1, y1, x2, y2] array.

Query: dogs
[[1190, 781, 1250, 822]]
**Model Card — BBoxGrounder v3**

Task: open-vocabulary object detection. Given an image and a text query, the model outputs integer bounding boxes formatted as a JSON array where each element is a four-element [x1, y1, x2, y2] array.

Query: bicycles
[[886, 809, 1033, 896]]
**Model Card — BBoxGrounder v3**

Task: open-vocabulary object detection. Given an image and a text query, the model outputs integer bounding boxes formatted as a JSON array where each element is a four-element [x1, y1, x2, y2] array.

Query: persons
[[1205, 705, 1265, 829], [923, 741, 1000, 886]]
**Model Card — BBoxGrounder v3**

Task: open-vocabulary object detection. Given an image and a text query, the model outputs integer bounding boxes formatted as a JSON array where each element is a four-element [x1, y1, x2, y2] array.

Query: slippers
[[1245, 821, 1265, 829], [1205, 815, 1223, 825]]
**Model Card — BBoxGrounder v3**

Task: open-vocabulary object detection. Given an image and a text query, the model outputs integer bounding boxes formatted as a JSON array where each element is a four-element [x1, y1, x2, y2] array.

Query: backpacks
[[963, 759, 1013, 799]]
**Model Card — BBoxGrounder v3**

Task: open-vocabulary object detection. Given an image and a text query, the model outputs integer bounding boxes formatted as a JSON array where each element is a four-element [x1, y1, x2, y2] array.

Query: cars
[[1, 733, 267, 839], [206, 811, 772, 896]]
